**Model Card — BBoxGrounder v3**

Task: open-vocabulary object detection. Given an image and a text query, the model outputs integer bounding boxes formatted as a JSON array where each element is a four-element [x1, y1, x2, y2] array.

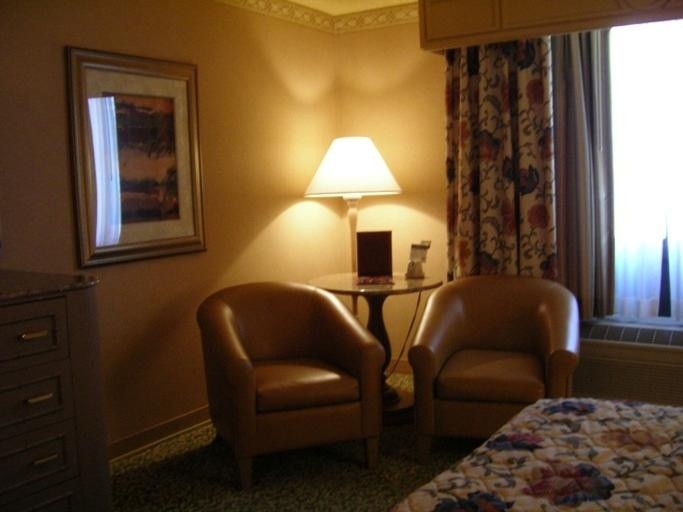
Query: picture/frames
[[63, 43, 211, 268]]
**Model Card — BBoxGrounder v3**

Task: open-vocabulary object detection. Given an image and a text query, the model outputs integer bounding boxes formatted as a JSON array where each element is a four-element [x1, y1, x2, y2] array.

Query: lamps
[[304, 138, 402, 324]]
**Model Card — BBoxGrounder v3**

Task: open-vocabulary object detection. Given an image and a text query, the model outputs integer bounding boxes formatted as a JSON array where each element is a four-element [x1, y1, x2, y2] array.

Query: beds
[[390, 398, 681, 510]]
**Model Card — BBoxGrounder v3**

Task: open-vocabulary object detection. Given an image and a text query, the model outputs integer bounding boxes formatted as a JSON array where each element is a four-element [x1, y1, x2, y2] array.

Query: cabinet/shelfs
[[0, 270, 110, 512], [418, 0, 683, 50]]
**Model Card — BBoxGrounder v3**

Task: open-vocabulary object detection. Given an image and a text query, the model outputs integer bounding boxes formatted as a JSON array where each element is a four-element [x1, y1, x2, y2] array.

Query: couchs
[[197, 282, 389, 488], [408, 277, 580, 462]]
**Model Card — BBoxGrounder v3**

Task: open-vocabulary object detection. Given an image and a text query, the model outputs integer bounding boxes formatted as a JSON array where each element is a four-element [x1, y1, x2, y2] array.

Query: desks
[[314, 275, 443, 407]]
